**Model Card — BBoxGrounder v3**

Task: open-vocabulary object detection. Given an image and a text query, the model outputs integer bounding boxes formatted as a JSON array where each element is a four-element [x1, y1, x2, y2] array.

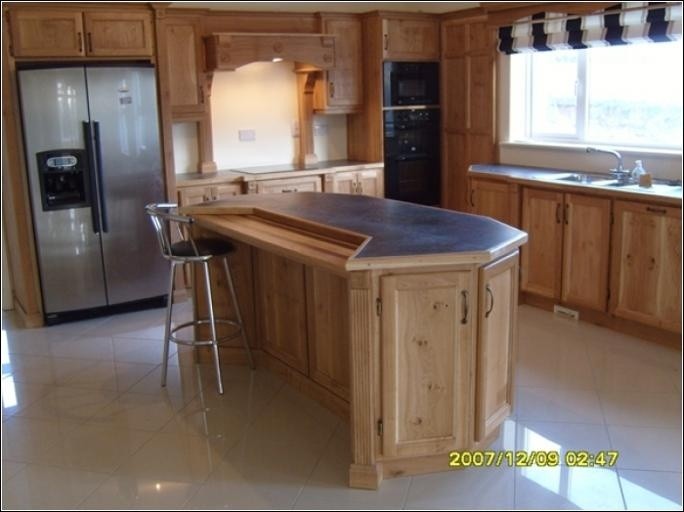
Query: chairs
[[145, 199, 257, 397]]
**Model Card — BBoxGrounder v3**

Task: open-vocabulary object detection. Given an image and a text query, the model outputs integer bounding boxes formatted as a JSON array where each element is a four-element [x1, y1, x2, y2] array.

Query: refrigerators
[[16, 58, 174, 324]]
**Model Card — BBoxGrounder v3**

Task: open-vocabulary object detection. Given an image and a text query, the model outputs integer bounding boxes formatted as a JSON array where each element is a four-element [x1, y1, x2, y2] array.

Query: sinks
[[557, 172, 610, 185]]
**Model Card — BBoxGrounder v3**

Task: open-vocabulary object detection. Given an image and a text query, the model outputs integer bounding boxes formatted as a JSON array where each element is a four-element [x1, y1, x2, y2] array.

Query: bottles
[[632, 160, 645, 184]]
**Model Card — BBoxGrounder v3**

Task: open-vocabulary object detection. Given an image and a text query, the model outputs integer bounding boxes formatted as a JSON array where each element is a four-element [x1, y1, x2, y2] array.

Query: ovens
[[383, 107, 442, 210]]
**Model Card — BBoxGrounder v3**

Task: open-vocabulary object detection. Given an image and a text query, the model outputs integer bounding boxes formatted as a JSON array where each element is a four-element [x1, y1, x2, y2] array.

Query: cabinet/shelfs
[[158, 8, 209, 123], [444, 12, 497, 213], [6, 4, 156, 61], [249, 175, 323, 194], [312, 12, 365, 114], [466, 180, 521, 227], [519, 188, 608, 328], [612, 199, 683, 351], [323, 168, 387, 199], [363, 12, 442, 60], [375, 251, 520, 459], [177, 182, 245, 207]]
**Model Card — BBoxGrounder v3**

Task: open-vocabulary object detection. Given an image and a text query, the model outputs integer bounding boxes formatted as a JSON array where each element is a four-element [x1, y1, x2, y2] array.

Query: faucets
[[586, 146, 631, 185]]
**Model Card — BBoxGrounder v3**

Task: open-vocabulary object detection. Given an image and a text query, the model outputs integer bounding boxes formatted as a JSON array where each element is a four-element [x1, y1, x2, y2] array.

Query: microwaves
[[382, 60, 440, 106]]
